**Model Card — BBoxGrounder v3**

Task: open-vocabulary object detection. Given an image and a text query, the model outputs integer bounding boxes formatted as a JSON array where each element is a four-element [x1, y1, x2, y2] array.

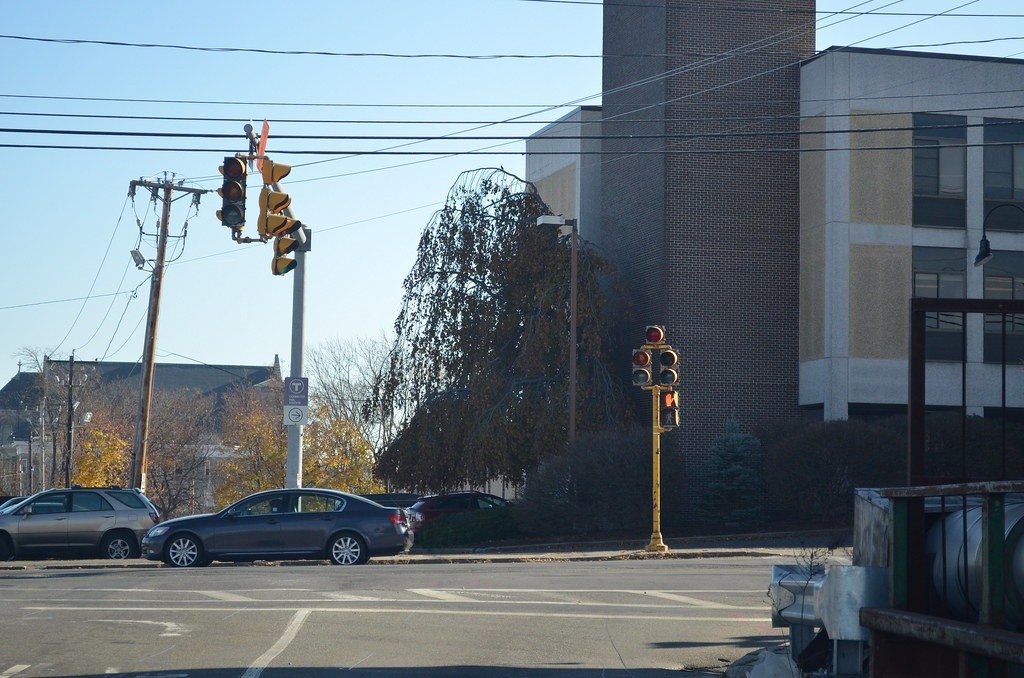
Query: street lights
[[534, 214, 578, 445]]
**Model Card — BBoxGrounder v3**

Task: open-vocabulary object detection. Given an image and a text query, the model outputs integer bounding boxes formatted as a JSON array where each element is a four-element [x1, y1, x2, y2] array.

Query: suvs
[[0, 485, 160, 562]]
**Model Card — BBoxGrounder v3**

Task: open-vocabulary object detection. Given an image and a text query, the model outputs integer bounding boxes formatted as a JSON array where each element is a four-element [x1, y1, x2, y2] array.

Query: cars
[[140, 488, 413, 567], [409, 487, 520, 548], [335, 492, 426, 512], [0, 493, 87, 516]]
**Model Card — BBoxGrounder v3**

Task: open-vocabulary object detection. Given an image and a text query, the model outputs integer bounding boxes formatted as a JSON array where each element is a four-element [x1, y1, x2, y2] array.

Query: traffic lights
[[216, 164, 222, 220], [256, 154, 289, 240], [633, 349, 651, 386], [270, 215, 303, 275], [659, 391, 679, 427], [660, 351, 680, 387], [221, 155, 246, 228], [645, 326, 666, 346]]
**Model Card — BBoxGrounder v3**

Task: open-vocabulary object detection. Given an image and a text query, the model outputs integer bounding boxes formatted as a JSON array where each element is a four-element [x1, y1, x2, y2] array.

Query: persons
[[267, 501, 280, 514]]
